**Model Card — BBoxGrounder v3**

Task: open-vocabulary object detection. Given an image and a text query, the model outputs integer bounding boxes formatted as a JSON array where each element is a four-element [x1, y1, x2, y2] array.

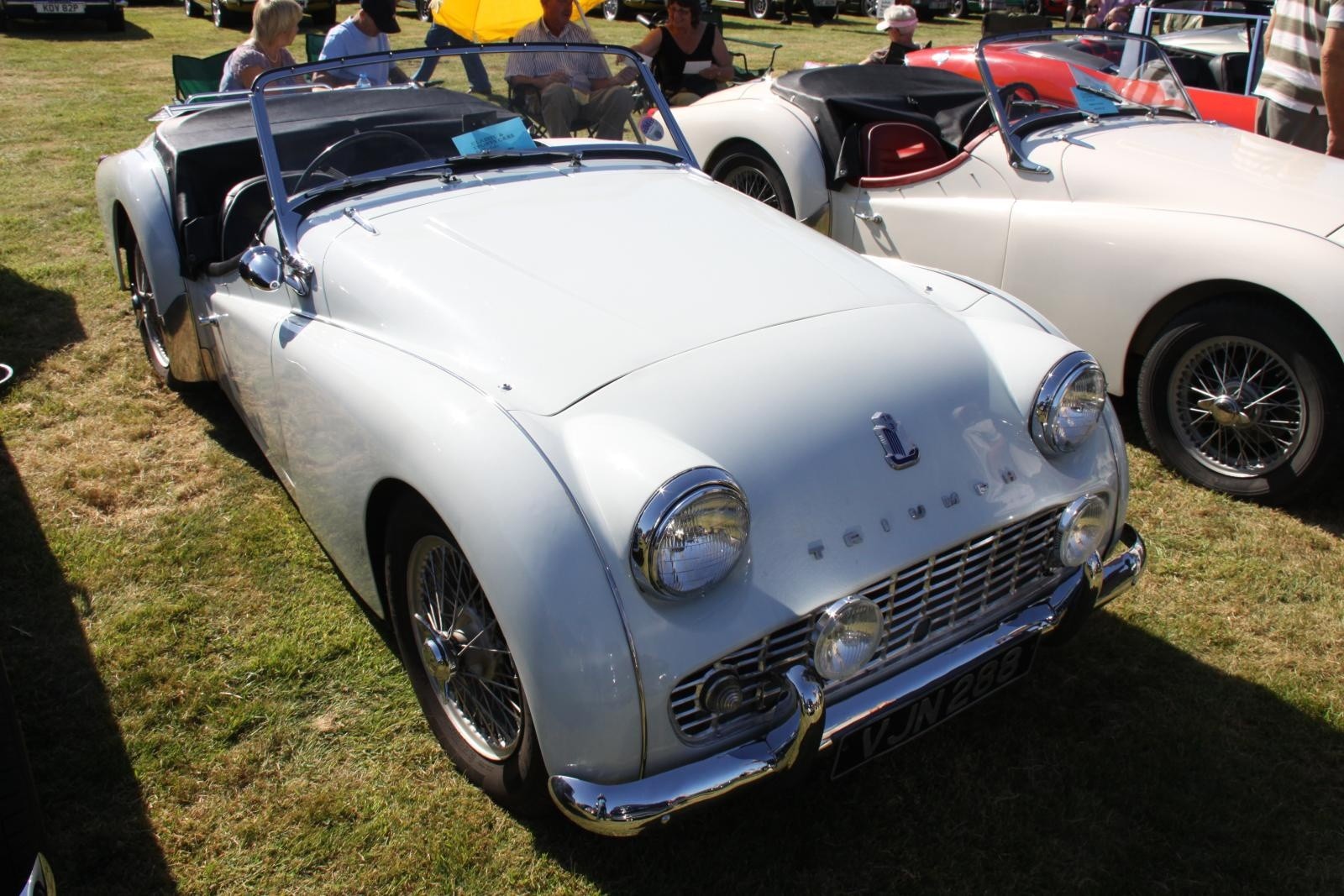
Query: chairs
[[172, 48, 237, 101], [303, 34, 329, 90], [505, 35, 782, 140], [884, 39, 935, 65], [1213, 48, 1256, 92], [856, 121, 947, 176], [1152, 57, 1210, 92]]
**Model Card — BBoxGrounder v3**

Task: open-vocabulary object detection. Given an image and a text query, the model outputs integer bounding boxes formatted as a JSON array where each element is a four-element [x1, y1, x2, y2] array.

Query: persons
[[219, 0, 312, 101], [413, 19, 496, 97], [1064, 0, 1140, 40], [504, 0, 641, 139], [1253, 0, 1344, 160], [312, 0, 426, 92], [616, 0, 735, 108], [857, 5, 926, 67]]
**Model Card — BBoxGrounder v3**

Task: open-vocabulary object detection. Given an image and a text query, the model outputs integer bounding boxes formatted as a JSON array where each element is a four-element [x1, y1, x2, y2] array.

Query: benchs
[[162, 95, 503, 219]]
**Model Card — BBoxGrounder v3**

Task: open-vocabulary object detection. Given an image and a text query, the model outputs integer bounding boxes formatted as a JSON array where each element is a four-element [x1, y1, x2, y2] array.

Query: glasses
[[669, 3, 694, 17]]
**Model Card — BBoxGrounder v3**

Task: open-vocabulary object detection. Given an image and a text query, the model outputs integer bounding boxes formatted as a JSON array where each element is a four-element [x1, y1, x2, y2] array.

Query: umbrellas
[[428, 0, 643, 144]]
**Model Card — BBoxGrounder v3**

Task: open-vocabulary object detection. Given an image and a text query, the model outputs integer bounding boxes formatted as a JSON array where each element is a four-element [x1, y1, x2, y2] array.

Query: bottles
[[356, 73, 371, 88]]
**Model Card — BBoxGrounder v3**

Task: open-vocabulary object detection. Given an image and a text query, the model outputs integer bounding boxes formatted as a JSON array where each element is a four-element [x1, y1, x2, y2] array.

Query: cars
[[643, 33, 1344, 500], [96, 42, 1150, 843], [904, 7, 1263, 132], [187, 0, 338, 30], [0, 0, 130, 27], [590, 0, 1085, 20]]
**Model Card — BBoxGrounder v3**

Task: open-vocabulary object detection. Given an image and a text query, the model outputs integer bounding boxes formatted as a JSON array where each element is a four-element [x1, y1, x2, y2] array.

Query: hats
[[360, 0, 401, 34], [875, 18, 920, 31]]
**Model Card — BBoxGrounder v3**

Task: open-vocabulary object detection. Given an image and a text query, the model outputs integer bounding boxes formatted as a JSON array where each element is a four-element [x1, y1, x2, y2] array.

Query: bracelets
[[716, 67, 721, 80]]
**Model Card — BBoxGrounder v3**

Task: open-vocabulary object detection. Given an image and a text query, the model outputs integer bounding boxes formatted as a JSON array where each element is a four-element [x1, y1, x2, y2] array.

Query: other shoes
[[812, 17, 828, 27], [778, 20, 791, 25]]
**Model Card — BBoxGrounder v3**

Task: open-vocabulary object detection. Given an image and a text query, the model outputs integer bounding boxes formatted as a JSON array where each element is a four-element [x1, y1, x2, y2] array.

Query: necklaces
[[262, 48, 279, 63]]
